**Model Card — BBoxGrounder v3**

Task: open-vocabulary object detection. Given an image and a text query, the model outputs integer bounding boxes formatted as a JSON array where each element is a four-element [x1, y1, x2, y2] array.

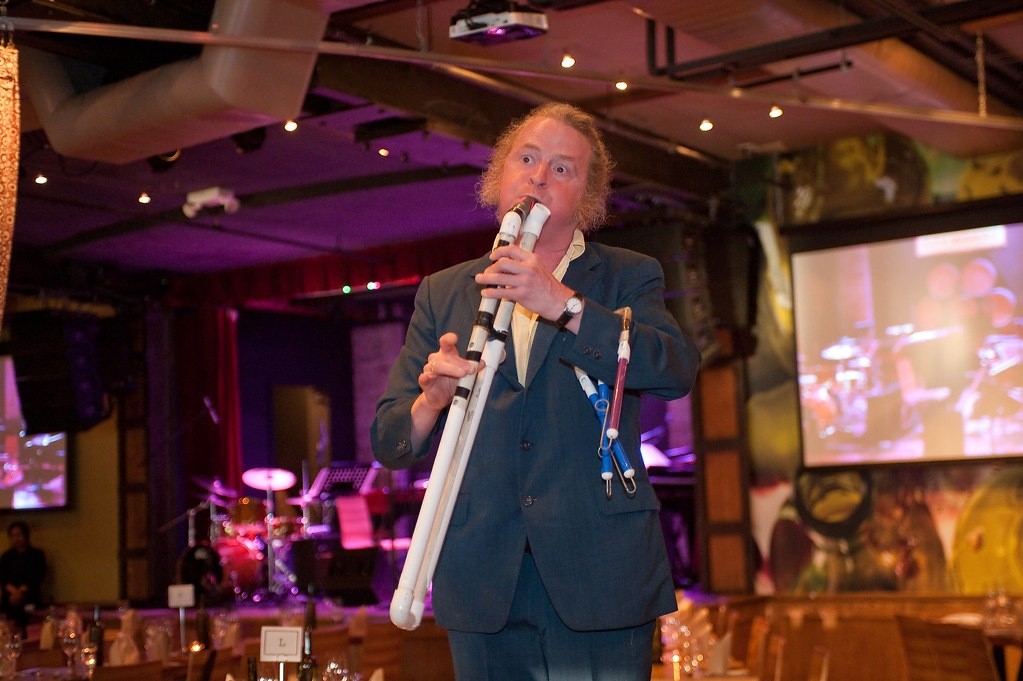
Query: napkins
[[109, 633, 140, 666], [659, 599, 732, 674], [40, 619, 54, 649], [120, 609, 137, 633], [222, 621, 240, 647]]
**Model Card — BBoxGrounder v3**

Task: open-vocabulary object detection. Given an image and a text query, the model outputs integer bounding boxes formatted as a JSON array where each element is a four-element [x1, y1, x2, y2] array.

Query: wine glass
[[2, 633, 22, 681], [59, 626, 79, 681], [80, 643, 96, 680]]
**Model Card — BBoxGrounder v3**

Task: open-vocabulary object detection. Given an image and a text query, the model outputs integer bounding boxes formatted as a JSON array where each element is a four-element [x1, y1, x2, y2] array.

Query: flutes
[[388, 196, 551, 631]]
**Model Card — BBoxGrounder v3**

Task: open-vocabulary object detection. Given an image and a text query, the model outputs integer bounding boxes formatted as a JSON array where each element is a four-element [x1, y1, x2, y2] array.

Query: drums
[[175, 516, 411, 605]]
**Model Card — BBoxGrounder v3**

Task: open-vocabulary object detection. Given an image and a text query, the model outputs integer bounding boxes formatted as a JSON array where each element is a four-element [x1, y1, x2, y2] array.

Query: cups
[[322, 656, 344, 681]]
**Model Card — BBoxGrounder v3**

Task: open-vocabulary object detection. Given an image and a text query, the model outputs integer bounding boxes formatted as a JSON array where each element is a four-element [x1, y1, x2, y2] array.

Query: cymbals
[[242, 467, 297, 491], [189, 474, 238, 508]]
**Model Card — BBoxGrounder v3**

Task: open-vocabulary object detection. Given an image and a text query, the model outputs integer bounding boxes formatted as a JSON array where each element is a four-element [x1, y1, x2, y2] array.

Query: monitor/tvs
[[0, 430, 74, 517]]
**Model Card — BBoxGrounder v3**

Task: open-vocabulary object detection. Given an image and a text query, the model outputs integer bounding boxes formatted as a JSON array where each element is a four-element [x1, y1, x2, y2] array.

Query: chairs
[[0, 602, 349, 681], [335, 496, 412, 550], [721, 591, 1023, 681]]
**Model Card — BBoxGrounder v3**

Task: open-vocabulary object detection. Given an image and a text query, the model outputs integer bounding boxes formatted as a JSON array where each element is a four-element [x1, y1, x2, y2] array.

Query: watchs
[[556, 290, 582, 328]]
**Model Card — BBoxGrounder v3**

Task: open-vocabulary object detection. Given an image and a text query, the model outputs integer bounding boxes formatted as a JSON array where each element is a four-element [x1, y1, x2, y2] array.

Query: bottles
[[90, 602, 105, 666], [298, 629, 317, 681], [196, 596, 210, 650], [247, 656, 258, 681]]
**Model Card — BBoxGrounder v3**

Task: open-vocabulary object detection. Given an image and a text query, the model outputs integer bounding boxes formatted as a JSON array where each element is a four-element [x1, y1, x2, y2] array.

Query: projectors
[[183, 188, 239, 219], [448, 5, 548, 48]]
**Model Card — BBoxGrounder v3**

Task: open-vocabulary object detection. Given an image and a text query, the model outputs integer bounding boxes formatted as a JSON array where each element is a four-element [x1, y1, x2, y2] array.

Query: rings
[[429, 360, 436, 371]]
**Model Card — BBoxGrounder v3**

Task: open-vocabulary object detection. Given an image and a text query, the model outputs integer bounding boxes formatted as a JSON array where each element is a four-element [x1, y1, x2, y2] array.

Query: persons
[[372, 102, 701, 681], [0, 521, 46, 639]]
